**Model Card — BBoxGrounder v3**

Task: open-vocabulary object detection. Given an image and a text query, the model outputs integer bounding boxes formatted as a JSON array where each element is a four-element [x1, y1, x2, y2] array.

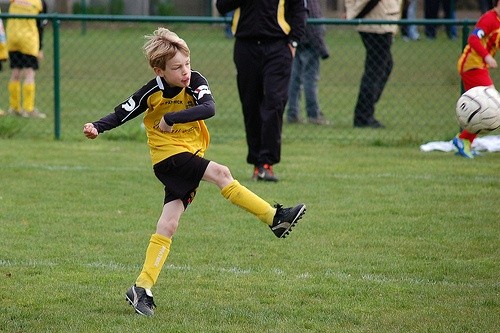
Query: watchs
[[289, 40, 298, 48]]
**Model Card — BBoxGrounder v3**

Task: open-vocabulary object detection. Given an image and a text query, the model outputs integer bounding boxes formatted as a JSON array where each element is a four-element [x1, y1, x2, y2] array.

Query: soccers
[[455, 86, 500, 136]]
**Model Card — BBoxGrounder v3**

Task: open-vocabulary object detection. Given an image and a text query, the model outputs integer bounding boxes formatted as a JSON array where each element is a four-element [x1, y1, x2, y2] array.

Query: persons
[[286, 0, 329, 124], [4, 0, 48, 119], [345, 0, 404, 126], [216, 0, 307, 181], [82, 27, 307, 317], [400, 0, 459, 40], [452, 0, 500, 159]]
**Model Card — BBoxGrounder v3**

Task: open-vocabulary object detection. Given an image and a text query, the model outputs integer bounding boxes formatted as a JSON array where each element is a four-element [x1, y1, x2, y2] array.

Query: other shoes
[[353, 120, 386, 129], [0, 107, 9, 117], [287, 117, 307, 127], [308, 117, 332, 126], [28, 108, 46, 119], [251, 163, 280, 183], [8, 106, 28, 118]]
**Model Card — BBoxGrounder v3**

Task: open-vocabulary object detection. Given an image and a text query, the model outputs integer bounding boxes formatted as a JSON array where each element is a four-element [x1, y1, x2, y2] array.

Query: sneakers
[[268, 202, 306, 239], [124, 284, 157, 318], [452, 134, 483, 159]]
